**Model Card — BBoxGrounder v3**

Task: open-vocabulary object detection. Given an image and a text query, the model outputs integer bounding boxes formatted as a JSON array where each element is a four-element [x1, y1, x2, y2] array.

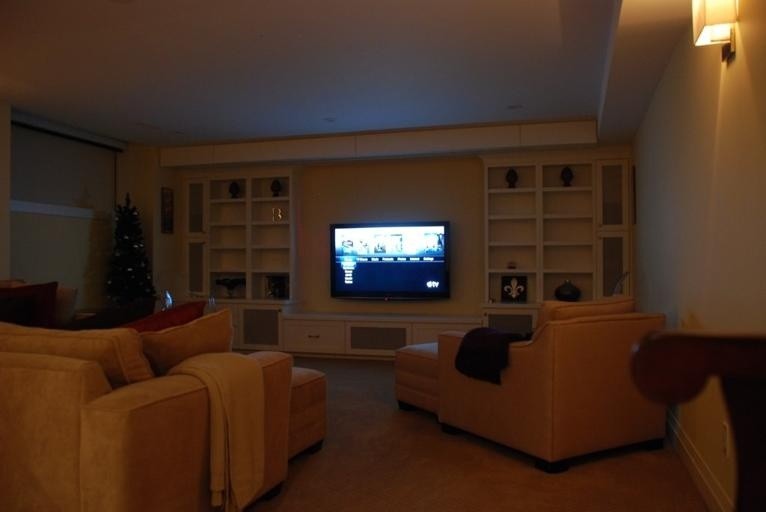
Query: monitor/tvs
[[330, 221, 450, 300]]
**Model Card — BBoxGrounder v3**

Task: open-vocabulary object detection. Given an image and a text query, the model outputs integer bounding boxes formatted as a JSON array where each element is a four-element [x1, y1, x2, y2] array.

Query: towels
[[167, 353, 266, 511]]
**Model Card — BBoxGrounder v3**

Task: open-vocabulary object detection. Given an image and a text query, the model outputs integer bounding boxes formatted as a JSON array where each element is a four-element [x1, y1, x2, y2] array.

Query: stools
[[287, 365, 326, 462], [394, 341, 438, 415]]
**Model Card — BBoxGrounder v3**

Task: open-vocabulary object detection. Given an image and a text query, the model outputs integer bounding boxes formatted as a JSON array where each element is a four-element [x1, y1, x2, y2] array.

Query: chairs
[[437, 297, 672, 475], [1, 344, 295, 510]]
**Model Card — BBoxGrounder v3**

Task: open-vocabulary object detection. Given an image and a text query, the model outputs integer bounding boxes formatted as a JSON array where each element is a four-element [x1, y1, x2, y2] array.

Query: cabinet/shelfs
[[183, 178, 206, 297], [206, 176, 296, 305], [481, 308, 538, 339], [484, 157, 596, 308], [206, 298, 283, 351], [596, 157, 631, 303], [284, 313, 484, 358]]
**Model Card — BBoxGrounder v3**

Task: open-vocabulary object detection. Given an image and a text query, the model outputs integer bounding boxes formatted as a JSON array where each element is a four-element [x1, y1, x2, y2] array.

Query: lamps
[[692, 1, 741, 62]]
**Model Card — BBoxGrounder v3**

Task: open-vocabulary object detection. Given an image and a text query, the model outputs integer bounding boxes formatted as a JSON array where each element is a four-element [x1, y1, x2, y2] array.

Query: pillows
[[1, 278, 76, 327], [110, 299, 208, 334], [139, 305, 239, 373]]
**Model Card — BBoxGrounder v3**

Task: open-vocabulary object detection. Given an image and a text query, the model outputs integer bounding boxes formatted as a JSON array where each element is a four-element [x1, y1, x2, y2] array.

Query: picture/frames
[[160, 187, 174, 233]]
